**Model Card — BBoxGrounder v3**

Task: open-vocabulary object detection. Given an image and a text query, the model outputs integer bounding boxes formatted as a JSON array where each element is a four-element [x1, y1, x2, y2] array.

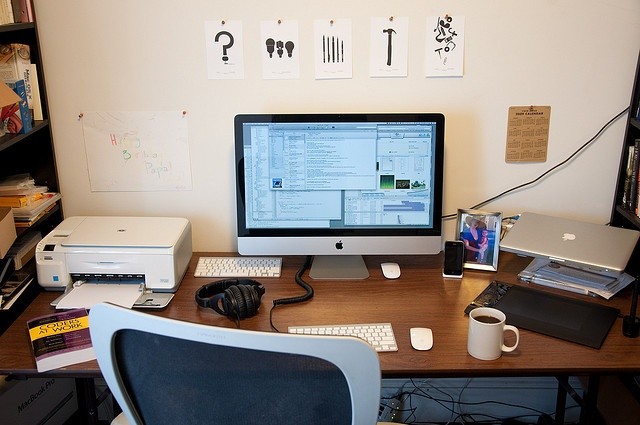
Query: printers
[[36, 215, 194, 309]]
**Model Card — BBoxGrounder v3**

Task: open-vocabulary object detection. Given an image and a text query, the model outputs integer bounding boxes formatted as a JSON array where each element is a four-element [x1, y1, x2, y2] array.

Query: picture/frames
[[455, 206, 502, 273]]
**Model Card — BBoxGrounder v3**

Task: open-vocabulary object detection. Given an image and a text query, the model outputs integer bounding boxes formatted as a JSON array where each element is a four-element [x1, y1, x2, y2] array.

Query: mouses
[[410, 326, 434, 351], [380, 263, 401, 279]]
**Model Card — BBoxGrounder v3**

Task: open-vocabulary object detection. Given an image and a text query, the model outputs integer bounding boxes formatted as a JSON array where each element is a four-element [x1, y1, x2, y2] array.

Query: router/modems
[[379, 398, 401, 423]]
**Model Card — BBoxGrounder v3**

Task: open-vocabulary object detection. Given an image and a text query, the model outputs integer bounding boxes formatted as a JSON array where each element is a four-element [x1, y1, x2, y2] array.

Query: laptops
[[500, 211, 640, 274]]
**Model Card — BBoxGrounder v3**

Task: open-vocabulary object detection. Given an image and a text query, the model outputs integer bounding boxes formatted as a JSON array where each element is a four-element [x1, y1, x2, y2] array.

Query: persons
[[479, 228, 487, 264], [461, 214, 487, 263]]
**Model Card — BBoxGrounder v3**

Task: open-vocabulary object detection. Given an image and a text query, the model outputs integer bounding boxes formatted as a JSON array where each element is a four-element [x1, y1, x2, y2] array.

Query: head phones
[[195, 278, 265, 320]]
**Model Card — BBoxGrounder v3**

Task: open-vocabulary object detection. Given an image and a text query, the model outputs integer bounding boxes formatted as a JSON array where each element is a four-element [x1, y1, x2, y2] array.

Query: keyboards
[[195, 256, 282, 279], [286, 322, 398, 353]]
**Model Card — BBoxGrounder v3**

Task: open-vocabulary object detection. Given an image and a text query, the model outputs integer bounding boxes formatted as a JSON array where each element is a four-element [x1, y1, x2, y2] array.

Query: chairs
[[89, 300, 410, 425]]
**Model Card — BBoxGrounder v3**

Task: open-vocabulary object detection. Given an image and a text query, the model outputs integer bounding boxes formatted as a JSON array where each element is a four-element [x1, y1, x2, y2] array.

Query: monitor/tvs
[[235, 112, 446, 280]]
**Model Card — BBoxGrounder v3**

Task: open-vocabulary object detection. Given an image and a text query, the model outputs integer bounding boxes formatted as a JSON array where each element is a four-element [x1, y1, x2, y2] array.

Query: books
[[0, 273, 34, 309], [620, 136, 640, 221], [1, 175, 63, 226], [0, 1, 33, 26], [28, 308, 96, 372], [0, 43, 43, 136]]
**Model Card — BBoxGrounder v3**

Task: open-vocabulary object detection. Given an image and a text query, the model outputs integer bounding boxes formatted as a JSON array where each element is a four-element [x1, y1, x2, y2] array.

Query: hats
[[465, 214, 487, 230]]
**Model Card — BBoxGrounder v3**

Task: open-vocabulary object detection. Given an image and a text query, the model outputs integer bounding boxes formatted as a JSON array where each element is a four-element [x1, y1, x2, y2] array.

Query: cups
[[468, 307, 520, 362]]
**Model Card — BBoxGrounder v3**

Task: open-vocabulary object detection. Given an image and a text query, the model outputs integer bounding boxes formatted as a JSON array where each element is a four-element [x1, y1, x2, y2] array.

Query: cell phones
[[444, 240, 465, 282]]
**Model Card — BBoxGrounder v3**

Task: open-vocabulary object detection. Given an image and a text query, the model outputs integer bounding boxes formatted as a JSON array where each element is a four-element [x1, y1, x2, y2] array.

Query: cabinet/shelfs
[[610, 55, 639, 228], [0, 0, 65, 336]]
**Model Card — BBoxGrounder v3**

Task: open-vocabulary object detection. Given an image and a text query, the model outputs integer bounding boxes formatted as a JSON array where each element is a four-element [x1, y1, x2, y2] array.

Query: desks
[[1, 250, 639, 425]]
